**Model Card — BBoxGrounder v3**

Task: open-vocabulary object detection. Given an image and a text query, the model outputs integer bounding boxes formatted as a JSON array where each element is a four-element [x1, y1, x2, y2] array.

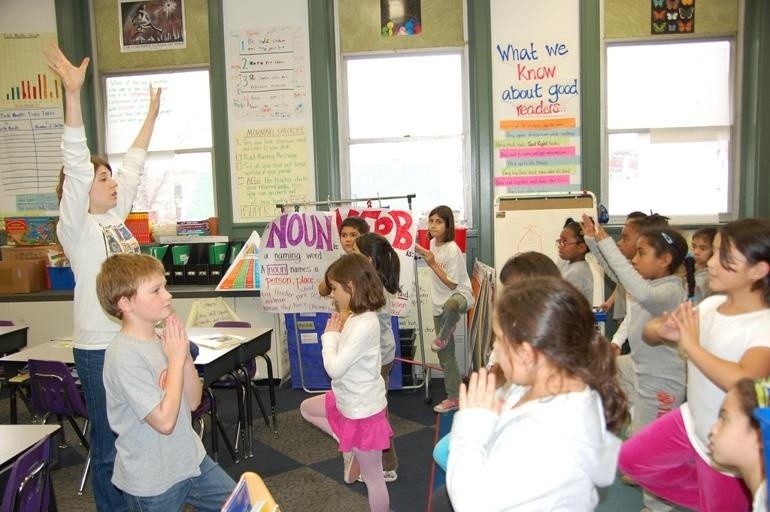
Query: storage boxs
[[0, 260, 46, 295], [49, 262, 76, 289], [2, 243, 67, 266], [399, 328, 420, 389]]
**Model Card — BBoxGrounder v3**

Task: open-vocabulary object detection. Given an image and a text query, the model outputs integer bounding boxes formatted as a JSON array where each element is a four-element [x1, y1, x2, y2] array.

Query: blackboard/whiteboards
[[494, 208, 605, 313]]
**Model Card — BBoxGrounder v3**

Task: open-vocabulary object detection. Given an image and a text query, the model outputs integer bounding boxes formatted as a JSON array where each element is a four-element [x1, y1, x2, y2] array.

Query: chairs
[[0, 321, 91, 511], [156, 321, 277, 467]]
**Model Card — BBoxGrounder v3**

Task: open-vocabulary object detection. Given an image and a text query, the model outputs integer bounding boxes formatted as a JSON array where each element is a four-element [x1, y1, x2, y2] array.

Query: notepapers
[[8, 374, 30, 383]]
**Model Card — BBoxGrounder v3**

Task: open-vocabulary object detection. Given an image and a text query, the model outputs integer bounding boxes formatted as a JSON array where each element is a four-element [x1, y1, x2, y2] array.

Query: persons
[[96, 251, 247, 510], [44, 39, 163, 509]]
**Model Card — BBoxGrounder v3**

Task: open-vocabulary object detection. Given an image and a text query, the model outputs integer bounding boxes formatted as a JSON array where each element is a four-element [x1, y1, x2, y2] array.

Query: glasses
[[557, 237, 583, 246]]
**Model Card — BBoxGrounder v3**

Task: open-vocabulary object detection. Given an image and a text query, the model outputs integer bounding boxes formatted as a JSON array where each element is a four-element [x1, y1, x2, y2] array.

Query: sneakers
[[431, 325, 457, 353], [430, 399, 460, 414], [356, 470, 397, 484], [341, 448, 360, 485], [622, 471, 654, 511]]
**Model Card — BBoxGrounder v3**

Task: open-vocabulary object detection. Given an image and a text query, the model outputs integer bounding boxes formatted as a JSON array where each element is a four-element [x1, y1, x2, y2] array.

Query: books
[[47, 248, 73, 267], [189, 332, 245, 349], [176, 220, 210, 237]]
